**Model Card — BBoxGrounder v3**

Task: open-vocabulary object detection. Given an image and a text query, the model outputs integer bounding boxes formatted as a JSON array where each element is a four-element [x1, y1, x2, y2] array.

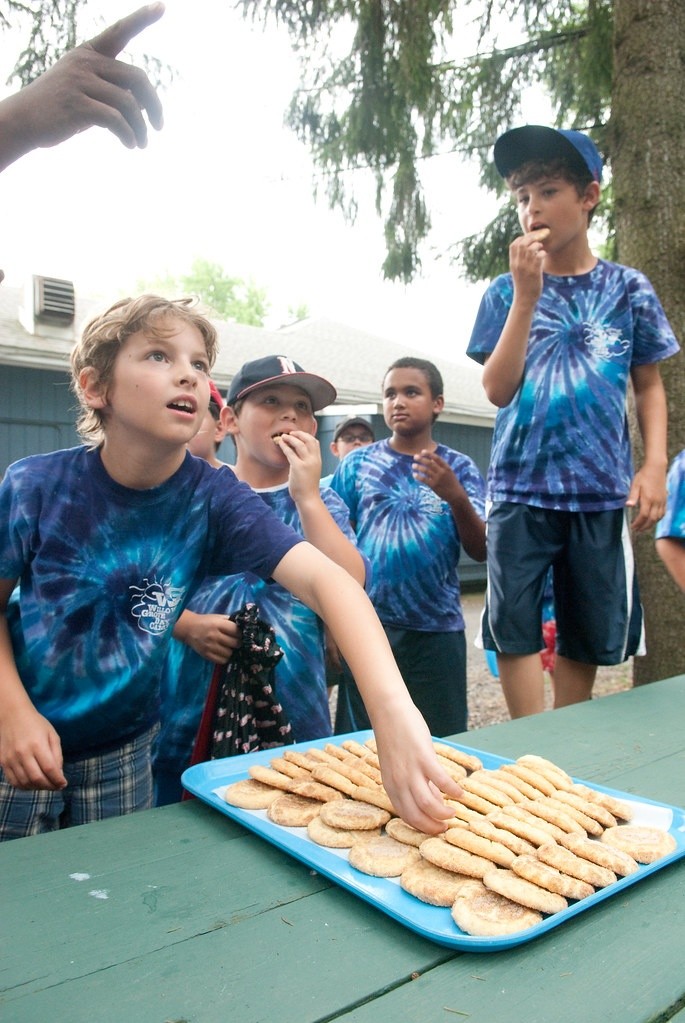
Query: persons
[[654, 450, 685, 592], [0, 294, 463, 864], [329, 356, 488, 737], [0, 1, 166, 173], [184, 381, 234, 470], [466, 125, 681, 721], [320, 415, 377, 486], [151, 354, 373, 806]]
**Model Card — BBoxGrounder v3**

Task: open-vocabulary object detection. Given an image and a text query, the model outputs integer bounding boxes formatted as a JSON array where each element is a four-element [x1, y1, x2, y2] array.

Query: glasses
[[335, 433, 374, 444]]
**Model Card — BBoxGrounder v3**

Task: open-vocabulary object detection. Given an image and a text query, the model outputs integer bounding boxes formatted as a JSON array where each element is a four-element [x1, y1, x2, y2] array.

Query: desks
[[0, 674, 685, 1023]]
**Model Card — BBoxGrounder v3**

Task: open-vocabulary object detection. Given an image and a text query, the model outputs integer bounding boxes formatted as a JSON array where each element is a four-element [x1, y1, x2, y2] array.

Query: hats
[[493, 125, 603, 214], [208, 379, 223, 411], [656, 450, 683, 536], [333, 416, 375, 443], [226, 354, 337, 413]]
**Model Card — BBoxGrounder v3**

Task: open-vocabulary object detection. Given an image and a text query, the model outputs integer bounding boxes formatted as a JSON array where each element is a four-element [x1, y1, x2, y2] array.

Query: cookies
[[528, 228, 552, 238], [221, 736, 676, 937]]
